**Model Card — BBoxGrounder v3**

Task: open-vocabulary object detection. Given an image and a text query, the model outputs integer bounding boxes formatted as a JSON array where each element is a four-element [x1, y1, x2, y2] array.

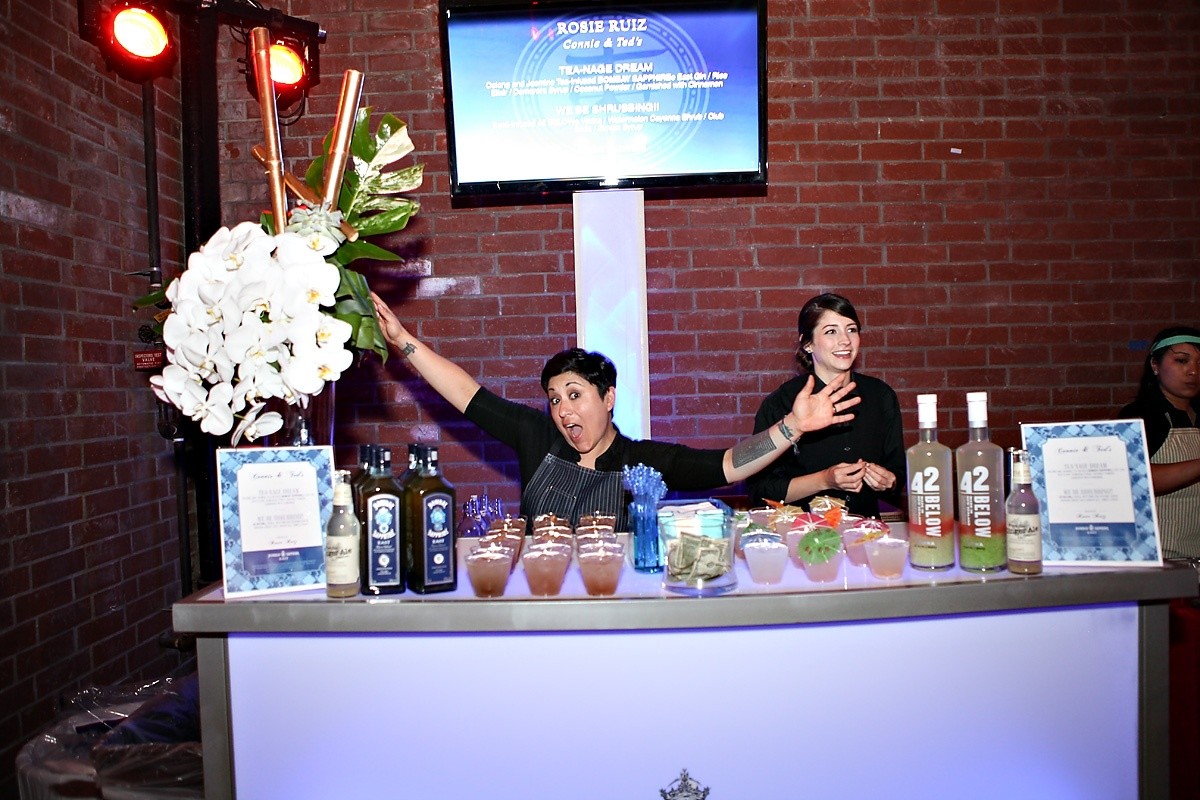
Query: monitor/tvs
[[438, 0, 770, 212]]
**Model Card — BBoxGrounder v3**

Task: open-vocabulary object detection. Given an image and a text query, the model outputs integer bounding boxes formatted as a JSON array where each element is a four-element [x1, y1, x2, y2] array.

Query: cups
[[734, 501, 910, 584], [465, 511, 624, 599]]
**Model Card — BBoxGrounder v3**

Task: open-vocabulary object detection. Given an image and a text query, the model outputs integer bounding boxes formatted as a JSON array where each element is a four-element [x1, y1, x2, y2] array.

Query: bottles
[[1005, 447, 1043, 576], [904, 393, 956, 572], [454, 490, 504, 540], [953, 392, 1008, 573], [325, 440, 460, 598]]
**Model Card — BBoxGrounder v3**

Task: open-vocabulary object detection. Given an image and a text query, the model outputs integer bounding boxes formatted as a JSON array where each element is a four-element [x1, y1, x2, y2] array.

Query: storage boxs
[[628, 499, 730, 575]]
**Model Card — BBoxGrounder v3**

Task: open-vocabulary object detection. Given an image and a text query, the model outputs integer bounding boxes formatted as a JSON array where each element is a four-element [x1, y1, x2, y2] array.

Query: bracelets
[[782, 415, 801, 454]]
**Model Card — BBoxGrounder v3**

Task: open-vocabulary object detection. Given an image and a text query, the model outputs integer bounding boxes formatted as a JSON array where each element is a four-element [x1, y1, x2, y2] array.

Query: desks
[[171, 522, 1200, 800]]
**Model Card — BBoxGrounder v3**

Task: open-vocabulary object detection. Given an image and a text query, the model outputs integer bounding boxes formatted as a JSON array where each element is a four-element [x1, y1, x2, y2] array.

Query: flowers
[[145, 106, 425, 445]]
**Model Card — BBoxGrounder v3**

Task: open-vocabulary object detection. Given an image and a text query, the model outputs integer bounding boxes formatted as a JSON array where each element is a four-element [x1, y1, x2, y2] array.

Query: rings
[[833, 405, 837, 413]]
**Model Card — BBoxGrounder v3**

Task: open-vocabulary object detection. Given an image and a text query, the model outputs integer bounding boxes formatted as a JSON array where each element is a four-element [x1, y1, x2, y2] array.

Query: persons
[[371, 291, 861, 535], [1115, 326, 1200, 559], [747, 293, 909, 521]]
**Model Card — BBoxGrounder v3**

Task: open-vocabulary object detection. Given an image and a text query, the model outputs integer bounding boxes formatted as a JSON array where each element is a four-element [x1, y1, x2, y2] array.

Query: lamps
[[267, 31, 310, 117], [113, 0, 174, 65]]
